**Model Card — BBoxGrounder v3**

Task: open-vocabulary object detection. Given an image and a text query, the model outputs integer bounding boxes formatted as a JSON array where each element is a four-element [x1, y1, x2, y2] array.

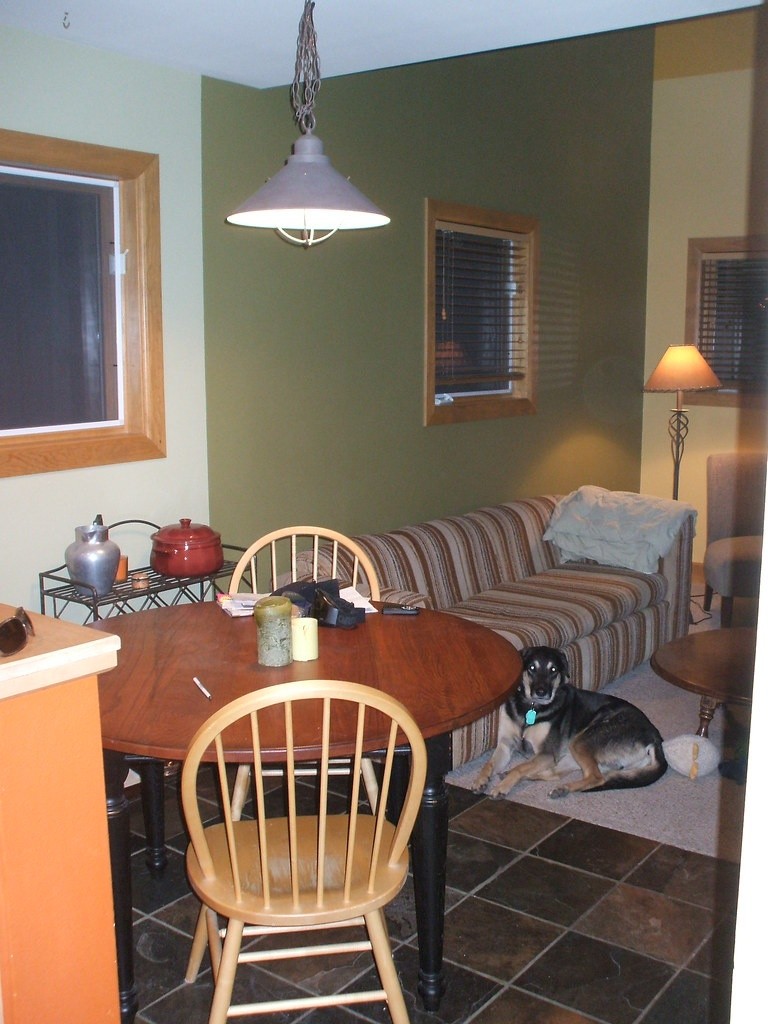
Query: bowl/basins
[[131, 573, 150, 591]]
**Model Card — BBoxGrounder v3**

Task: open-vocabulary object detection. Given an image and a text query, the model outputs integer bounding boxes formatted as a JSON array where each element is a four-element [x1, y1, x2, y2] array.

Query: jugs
[[64, 514, 121, 596]]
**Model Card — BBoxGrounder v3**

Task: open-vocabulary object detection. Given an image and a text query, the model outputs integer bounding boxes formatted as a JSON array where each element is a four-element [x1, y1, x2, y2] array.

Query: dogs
[[470, 645, 668, 802]]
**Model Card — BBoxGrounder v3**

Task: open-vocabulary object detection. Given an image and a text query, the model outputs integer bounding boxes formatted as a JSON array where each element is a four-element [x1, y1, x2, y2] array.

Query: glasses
[[0, 607, 36, 656]]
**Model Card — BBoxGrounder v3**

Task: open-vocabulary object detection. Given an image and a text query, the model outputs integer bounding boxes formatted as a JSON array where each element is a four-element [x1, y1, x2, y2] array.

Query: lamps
[[641, 344, 726, 505], [226, 3, 390, 244]]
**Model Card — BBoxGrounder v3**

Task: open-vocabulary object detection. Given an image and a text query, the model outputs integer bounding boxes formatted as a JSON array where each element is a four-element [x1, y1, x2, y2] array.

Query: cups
[[112, 555, 128, 584], [291, 618, 319, 661]]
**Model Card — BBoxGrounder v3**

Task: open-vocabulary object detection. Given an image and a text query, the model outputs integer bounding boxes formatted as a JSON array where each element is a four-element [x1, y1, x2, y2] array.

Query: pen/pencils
[[193, 676, 213, 700]]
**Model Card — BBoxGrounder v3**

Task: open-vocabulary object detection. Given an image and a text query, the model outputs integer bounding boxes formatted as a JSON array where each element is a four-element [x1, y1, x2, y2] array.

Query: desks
[[649, 627, 758, 742], [1, 598, 122, 1023], [80, 599, 522, 1012]]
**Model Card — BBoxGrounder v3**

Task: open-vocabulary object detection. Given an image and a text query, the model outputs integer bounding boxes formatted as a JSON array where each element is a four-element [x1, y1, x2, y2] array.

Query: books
[[215, 593, 276, 618]]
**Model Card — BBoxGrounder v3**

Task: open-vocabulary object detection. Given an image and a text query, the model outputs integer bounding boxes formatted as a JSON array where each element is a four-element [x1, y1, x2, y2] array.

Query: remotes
[[382, 606, 420, 615]]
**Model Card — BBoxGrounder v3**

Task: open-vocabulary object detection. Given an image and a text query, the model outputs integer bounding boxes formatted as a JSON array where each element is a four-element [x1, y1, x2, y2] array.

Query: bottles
[[253, 595, 293, 667]]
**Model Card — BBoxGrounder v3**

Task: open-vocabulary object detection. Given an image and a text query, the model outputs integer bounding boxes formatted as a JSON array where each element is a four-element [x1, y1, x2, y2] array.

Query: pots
[[149, 518, 225, 577]]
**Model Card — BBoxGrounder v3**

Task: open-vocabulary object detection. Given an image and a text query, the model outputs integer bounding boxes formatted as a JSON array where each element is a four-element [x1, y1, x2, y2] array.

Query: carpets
[[443, 564, 756, 864]]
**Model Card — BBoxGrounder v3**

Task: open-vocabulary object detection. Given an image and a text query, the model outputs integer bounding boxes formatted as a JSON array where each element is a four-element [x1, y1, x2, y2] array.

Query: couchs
[[286, 489, 694, 780]]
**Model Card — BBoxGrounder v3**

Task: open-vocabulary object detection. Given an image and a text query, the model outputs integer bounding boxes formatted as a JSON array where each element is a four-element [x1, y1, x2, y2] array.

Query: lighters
[[381, 607, 421, 615]]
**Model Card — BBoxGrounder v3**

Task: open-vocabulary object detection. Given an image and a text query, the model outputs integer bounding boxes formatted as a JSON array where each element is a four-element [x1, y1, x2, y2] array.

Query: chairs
[[702, 449, 768, 628], [176, 679, 428, 1024], [228, 525, 384, 831]]
